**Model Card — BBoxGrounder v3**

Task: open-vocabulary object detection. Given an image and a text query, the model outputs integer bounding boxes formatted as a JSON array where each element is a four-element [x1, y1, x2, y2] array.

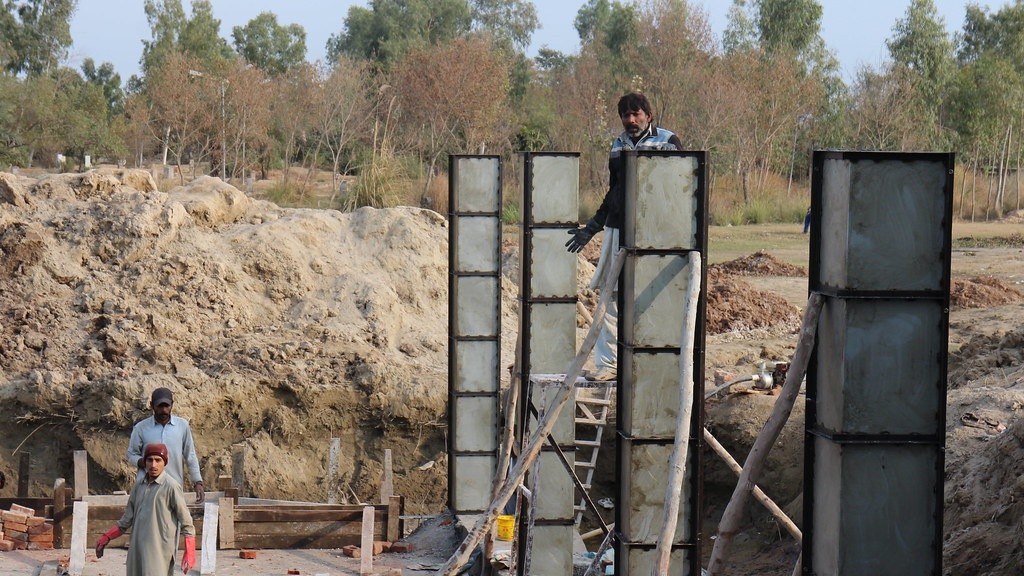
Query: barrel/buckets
[[496, 515, 515, 541]]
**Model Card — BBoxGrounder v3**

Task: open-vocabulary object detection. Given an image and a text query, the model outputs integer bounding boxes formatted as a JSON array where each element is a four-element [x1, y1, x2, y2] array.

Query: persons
[[802, 207, 812, 234], [564, 92, 685, 384], [95, 442, 197, 576], [126, 387, 206, 504]]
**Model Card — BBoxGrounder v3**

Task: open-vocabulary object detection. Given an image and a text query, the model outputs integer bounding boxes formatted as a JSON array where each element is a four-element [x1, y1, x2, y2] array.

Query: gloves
[[181, 535, 197, 575], [96, 524, 123, 558], [565, 219, 605, 253]]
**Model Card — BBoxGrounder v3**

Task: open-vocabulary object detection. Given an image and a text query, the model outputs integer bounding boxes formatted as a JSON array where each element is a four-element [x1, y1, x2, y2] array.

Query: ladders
[[572, 386, 613, 532]]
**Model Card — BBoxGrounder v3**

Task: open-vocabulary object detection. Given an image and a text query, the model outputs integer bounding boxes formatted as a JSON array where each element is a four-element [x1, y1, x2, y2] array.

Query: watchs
[[192, 481, 203, 489]]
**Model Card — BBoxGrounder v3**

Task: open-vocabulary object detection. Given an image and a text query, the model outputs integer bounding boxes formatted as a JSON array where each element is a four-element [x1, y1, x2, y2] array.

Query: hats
[[143, 442, 168, 467], [152, 387, 172, 406]]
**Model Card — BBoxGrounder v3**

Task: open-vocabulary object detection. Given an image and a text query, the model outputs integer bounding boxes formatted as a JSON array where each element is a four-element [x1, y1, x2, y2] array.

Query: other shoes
[[585, 367, 618, 381]]
